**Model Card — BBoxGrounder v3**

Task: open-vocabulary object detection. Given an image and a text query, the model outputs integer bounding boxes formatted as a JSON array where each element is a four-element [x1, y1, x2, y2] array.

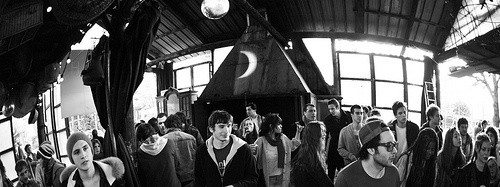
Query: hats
[[67, 133, 94, 164], [359, 120, 390, 148], [38, 143, 55, 160]]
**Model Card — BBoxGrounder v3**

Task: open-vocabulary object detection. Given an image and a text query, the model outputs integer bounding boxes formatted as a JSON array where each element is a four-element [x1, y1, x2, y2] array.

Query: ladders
[[422, 80, 439, 111]]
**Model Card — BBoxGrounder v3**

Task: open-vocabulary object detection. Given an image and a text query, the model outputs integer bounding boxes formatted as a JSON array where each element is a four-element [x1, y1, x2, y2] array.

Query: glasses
[[20, 169, 28, 177], [376, 141, 399, 152]]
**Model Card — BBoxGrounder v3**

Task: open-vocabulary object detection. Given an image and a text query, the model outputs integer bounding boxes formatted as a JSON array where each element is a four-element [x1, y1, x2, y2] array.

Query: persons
[[395, 128, 438, 187], [24, 144, 38, 176], [436, 128, 466, 187], [459, 134, 491, 187], [231, 103, 266, 144], [289, 103, 318, 164], [419, 104, 443, 153], [248, 114, 305, 187], [486, 127, 500, 168], [91, 138, 105, 160], [289, 121, 335, 187], [338, 105, 366, 167], [487, 156, 500, 187], [474, 120, 488, 138], [35, 143, 66, 187], [362, 107, 368, 124], [89, 129, 104, 146], [135, 111, 204, 187], [334, 119, 401, 187], [389, 100, 419, 165], [322, 98, 353, 185], [195, 110, 258, 187], [60, 132, 125, 187], [457, 118, 473, 163], [15, 160, 40, 187]]
